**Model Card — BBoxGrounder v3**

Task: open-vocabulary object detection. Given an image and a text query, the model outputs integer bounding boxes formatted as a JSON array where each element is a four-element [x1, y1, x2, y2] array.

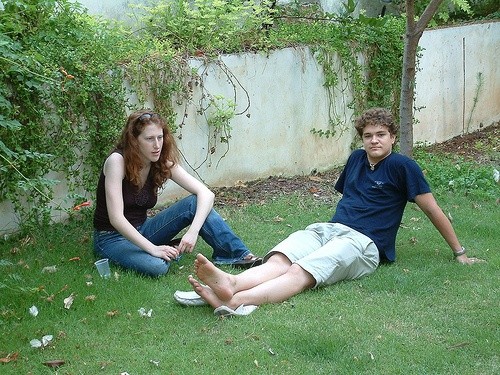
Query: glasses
[[130, 113, 160, 126]]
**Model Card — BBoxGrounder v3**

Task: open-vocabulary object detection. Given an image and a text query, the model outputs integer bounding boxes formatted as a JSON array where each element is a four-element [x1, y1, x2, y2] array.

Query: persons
[[188, 107, 485, 313], [93, 109, 264, 277]]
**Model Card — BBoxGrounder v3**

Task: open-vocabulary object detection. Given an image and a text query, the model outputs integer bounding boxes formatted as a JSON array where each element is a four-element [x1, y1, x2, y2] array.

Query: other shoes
[[214, 303, 257, 317], [174, 285, 212, 308]]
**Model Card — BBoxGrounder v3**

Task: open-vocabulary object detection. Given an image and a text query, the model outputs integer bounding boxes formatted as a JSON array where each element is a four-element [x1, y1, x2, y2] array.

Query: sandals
[[233, 252, 262, 265]]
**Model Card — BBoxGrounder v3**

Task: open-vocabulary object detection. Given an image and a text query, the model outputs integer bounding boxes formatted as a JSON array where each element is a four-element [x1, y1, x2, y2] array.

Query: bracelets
[[454, 246, 466, 256]]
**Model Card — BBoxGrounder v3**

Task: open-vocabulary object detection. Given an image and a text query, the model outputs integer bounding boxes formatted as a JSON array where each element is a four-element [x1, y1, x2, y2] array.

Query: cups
[[94, 258, 112, 280]]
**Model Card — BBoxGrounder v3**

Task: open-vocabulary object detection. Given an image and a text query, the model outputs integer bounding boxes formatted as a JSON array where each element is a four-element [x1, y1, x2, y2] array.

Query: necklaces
[[367, 152, 393, 171]]
